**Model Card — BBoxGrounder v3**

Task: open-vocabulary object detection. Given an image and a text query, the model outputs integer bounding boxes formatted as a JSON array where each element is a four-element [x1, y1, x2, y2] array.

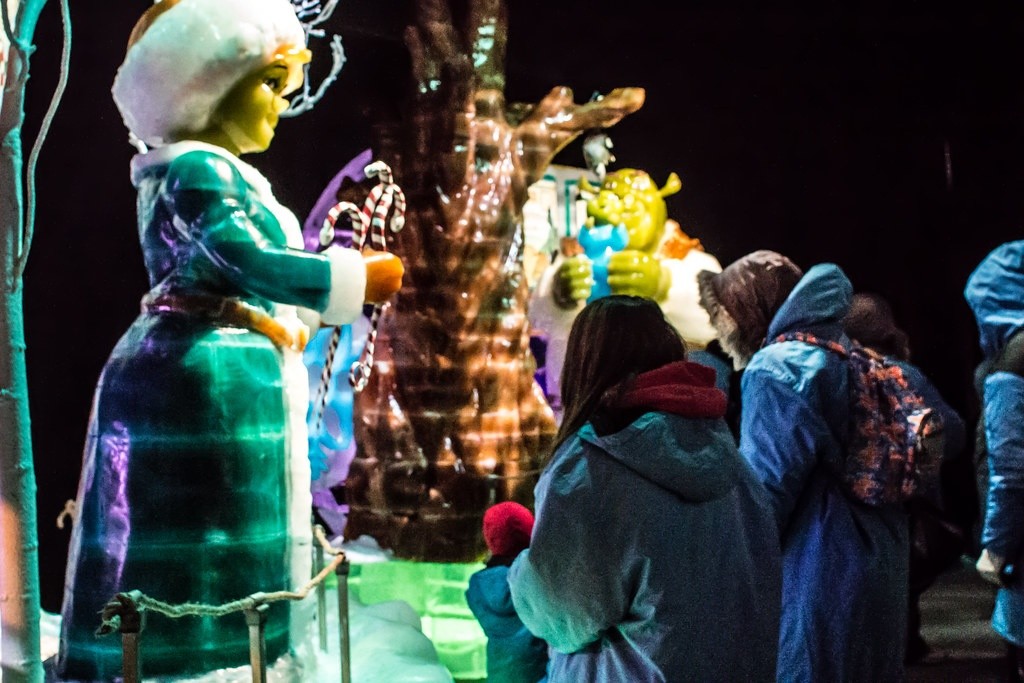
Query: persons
[[56, 0, 404, 683], [468, 238, 1024, 683]]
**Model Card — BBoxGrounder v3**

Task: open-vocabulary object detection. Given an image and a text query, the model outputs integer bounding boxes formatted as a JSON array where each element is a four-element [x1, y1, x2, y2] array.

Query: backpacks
[[764, 329, 941, 513]]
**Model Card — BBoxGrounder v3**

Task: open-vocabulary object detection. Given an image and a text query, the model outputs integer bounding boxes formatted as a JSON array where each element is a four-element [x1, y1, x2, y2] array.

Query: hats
[[483, 501, 535, 554]]
